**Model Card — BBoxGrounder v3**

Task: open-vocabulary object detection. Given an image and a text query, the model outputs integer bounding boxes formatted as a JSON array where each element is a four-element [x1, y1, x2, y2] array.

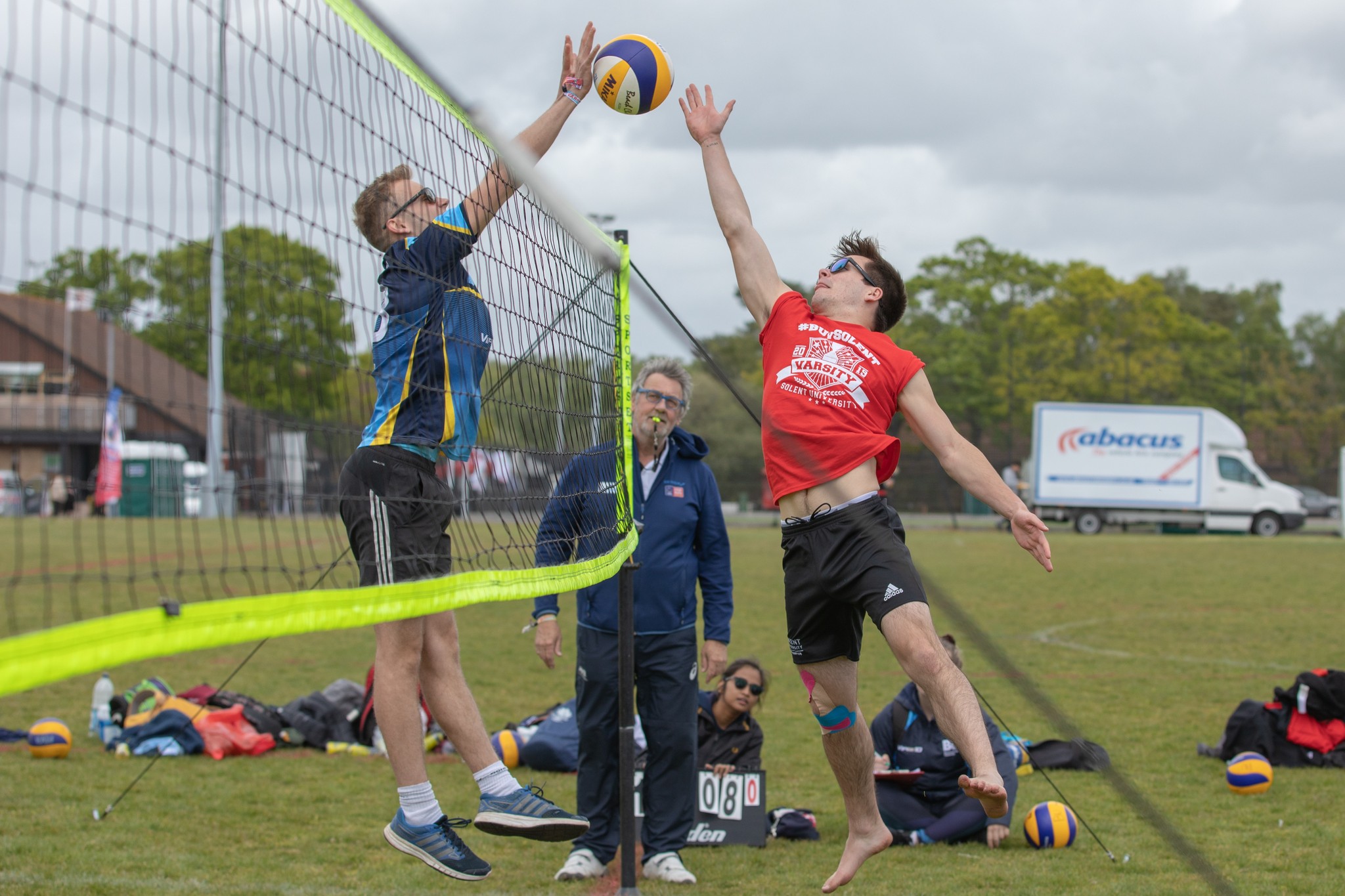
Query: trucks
[[1026, 397, 1310, 536]]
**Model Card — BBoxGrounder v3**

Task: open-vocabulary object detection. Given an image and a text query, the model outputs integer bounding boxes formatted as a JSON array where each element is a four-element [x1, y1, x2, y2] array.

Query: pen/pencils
[[874, 750, 890, 767]]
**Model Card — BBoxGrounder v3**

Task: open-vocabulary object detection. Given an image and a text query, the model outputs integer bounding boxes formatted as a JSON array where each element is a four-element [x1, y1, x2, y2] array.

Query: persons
[[337, 21, 601, 882], [990, 458, 1031, 529], [678, 84, 1053, 894], [869, 634, 1019, 849], [633, 659, 768, 778], [534, 358, 735, 887], [50, 469, 76, 518]]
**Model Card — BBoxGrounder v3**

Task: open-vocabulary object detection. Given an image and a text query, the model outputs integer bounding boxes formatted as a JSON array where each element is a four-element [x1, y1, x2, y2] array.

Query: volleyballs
[[28, 717, 71, 758], [594, 34, 675, 115], [1225, 749, 1273, 794], [488, 729, 526, 767], [1023, 801, 1078, 850]]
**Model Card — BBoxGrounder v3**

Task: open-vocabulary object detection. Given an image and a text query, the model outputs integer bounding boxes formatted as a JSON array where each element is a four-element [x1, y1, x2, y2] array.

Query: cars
[[1294, 483, 1342, 520]]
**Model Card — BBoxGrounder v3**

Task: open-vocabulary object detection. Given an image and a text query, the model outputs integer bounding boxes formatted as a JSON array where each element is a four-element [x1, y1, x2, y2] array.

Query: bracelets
[[563, 77, 583, 106], [519, 614, 558, 634]]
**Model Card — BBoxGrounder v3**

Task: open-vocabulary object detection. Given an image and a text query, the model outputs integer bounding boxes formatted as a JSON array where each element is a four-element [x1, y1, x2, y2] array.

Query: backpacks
[[768, 807, 821, 840], [356, 664, 433, 747], [1221, 699, 1277, 764], [521, 697, 579, 771]]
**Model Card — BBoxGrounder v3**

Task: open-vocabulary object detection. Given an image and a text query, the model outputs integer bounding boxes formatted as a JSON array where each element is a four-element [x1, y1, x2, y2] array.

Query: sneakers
[[642, 851, 698, 884], [384, 807, 493, 881], [473, 777, 591, 842], [554, 848, 613, 881]]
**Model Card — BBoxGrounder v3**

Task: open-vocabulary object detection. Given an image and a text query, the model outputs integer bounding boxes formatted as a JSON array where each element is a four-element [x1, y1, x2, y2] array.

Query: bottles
[[87, 673, 115, 737]]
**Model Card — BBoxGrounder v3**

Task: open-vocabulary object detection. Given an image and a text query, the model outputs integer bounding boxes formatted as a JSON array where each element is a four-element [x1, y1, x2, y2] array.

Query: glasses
[[725, 676, 764, 695], [825, 257, 886, 308], [636, 388, 685, 411], [382, 187, 436, 229]]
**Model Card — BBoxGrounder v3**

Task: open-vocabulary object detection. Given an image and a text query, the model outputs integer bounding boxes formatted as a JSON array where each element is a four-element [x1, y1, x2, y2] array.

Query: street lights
[[97, 308, 115, 390]]
